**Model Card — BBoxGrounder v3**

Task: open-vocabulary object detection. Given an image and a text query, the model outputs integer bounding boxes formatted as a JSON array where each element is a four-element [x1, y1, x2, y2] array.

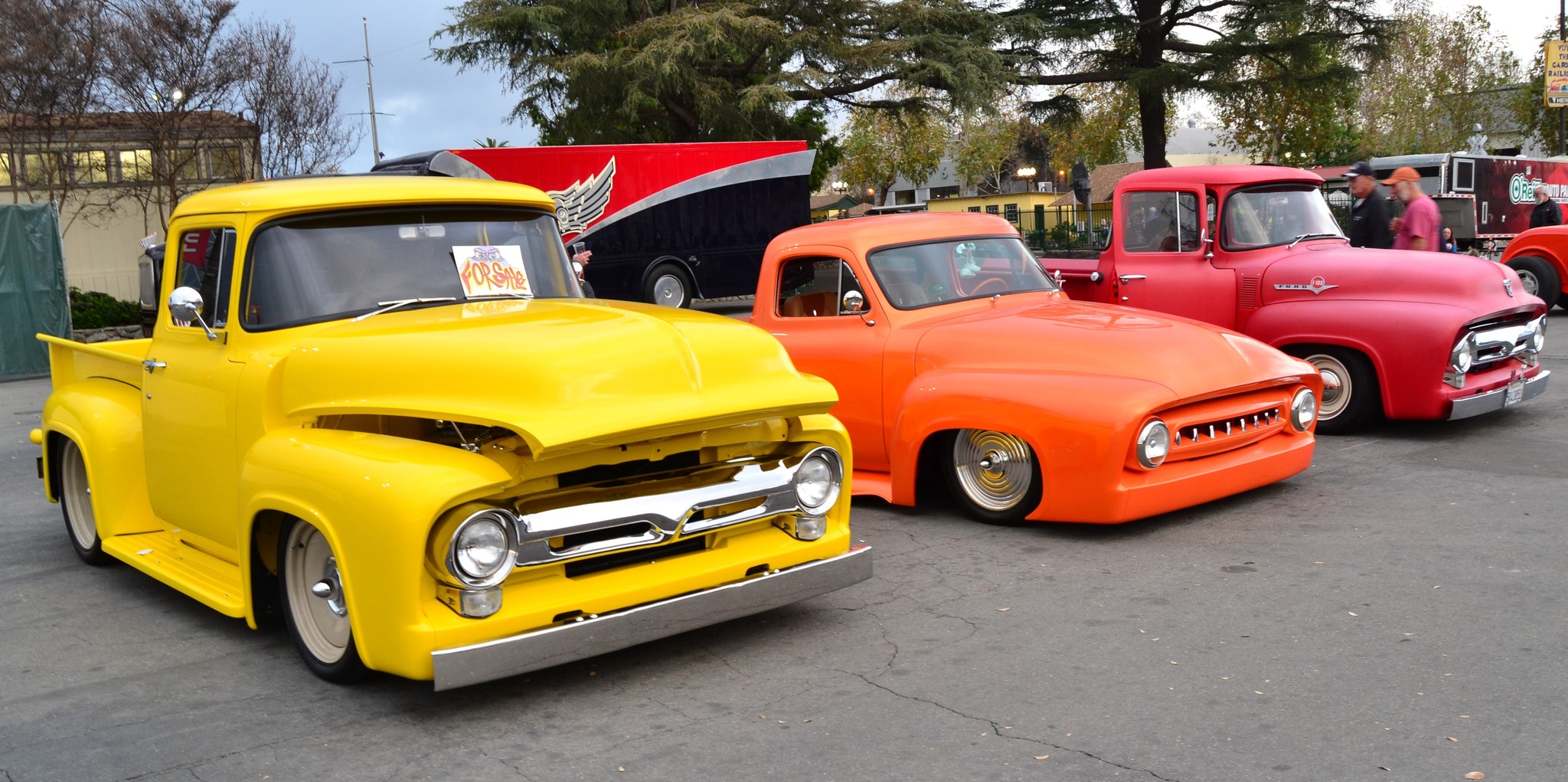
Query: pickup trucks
[[953, 163, 1553, 432], [29, 174, 876, 691], [723, 209, 1324, 528]]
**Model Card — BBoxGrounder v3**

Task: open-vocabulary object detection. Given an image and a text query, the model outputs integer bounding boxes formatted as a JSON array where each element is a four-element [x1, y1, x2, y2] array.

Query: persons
[[502, 221, 593, 296], [1439, 227, 1457, 254], [1529, 185, 1563, 230], [1340, 161, 1394, 250], [1380, 167, 1441, 252], [954, 242, 981, 279]]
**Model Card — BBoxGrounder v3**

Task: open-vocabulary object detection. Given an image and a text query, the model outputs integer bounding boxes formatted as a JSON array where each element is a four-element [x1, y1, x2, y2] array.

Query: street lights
[[1060, 170, 1067, 192], [830, 180, 849, 217]]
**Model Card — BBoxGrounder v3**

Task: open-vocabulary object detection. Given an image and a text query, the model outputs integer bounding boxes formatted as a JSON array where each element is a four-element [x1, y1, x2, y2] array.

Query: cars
[[1498, 222, 1568, 312]]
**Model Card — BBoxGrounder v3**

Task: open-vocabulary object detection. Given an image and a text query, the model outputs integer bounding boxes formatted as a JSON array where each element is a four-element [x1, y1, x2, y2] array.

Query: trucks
[[1366, 152, 1568, 254], [364, 140, 820, 309]]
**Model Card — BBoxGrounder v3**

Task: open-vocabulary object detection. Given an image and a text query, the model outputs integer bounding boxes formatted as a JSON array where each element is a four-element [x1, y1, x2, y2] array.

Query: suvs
[[135, 242, 166, 338]]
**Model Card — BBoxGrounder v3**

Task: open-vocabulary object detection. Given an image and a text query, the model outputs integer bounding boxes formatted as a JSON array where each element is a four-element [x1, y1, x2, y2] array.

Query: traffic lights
[[1016, 167, 1037, 192]]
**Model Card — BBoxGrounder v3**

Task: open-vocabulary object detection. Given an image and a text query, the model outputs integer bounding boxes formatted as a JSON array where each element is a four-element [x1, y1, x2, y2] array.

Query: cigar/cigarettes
[[588, 253, 592, 255]]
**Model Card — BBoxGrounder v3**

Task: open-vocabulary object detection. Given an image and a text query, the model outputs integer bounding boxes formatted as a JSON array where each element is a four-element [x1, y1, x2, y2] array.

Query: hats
[[1380, 168, 1420, 186], [1341, 162, 1374, 177]]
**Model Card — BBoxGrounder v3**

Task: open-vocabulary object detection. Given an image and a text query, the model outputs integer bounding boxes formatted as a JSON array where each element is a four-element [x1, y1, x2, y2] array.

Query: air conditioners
[[1038, 182, 1052, 192]]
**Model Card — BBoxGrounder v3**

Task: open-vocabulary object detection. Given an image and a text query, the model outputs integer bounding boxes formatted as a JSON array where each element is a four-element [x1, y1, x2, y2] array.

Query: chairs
[[783, 291, 846, 316], [883, 282, 929, 306]]
